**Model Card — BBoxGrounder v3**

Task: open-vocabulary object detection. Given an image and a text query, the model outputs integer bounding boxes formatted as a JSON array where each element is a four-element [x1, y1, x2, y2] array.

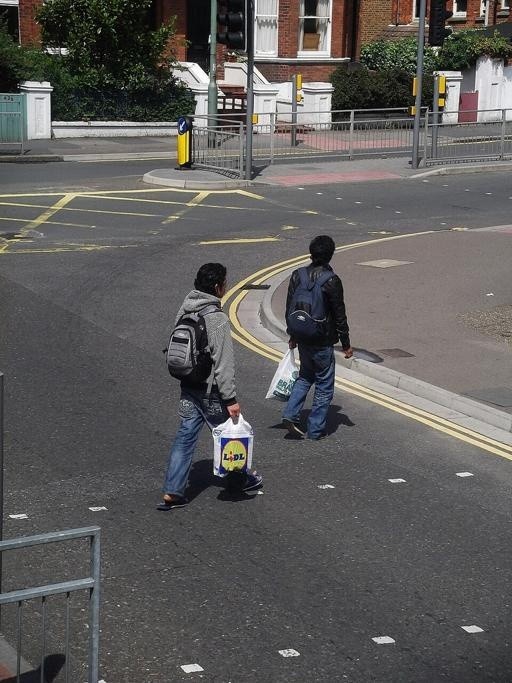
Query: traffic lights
[[430, 0, 454, 45], [225, 0, 248, 55], [217, 0, 227, 44]]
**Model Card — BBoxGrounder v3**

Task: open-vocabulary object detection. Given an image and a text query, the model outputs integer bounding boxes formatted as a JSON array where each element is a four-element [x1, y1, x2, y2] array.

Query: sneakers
[[283, 418, 305, 435]]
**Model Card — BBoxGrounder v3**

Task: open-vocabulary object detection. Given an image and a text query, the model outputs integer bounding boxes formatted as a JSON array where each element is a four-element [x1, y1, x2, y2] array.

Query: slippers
[[156, 495, 191, 509], [240, 474, 262, 491]]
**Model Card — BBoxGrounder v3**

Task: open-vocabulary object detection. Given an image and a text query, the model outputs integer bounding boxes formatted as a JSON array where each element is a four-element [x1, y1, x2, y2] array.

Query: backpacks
[[288, 266, 335, 340], [167, 304, 223, 382]]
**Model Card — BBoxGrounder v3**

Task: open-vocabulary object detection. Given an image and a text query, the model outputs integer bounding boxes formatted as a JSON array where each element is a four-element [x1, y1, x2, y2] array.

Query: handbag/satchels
[[212, 413, 253, 478]]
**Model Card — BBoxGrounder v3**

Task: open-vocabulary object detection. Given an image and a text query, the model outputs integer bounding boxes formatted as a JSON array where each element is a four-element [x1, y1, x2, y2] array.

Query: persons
[[161, 263, 263, 505], [284, 233, 354, 441]]
